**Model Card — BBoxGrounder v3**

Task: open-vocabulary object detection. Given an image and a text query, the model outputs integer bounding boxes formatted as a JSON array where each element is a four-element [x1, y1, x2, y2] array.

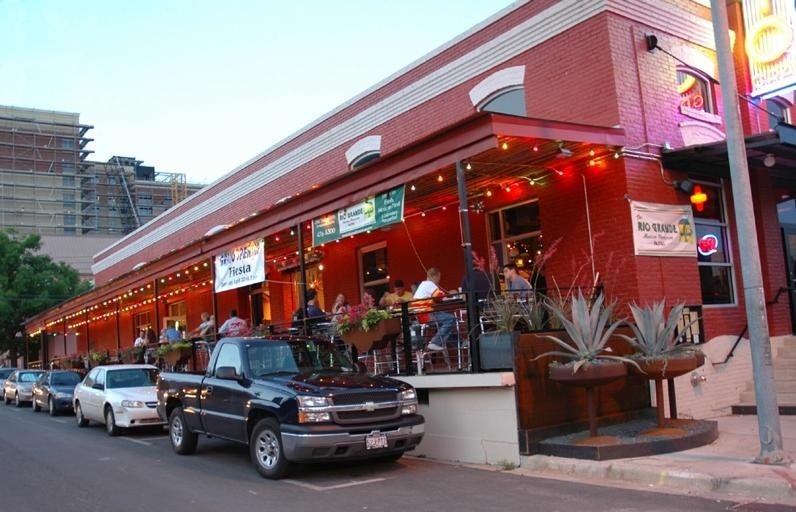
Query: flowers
[[470, 236, 626, 344]]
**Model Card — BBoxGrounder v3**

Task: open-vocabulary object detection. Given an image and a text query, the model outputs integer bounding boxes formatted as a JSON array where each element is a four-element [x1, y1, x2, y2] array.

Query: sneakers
[[428, 342, 445, 352]]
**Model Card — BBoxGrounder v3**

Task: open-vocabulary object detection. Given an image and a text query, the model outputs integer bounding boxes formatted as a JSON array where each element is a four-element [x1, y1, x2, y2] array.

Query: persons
[[332, 294, 351, 315], [218, 310, 247, 336], [188, 312, 214, 335], [291, 288, 330, 335], [134, 320, 182, 366], [379, 279, 413, 305], [412, 268, 455, 351], [503, 262, 534, 303], [462, 260, 501, 332]]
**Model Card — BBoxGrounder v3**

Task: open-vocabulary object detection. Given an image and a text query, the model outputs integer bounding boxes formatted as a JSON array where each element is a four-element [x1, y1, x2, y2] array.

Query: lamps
[[673, 180, 694, 191], [763, 153, 776, 167]]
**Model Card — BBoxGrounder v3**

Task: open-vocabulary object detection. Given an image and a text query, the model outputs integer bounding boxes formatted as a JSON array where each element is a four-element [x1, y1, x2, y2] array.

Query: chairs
[[286, 295, 534, 375]]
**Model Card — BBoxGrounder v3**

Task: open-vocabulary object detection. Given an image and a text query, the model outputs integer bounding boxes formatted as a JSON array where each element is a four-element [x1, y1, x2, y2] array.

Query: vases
[[478, 330, 521, 371]]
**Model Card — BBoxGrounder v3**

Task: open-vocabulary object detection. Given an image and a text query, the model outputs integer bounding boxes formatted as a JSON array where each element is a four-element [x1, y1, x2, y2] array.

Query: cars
[[0, 368, 80, 417], [73, 365, 170, 434]]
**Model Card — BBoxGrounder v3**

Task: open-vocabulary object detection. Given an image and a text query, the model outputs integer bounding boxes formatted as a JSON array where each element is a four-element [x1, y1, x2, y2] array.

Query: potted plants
[[530, 286, 649, 448], [614, 296, 698, 439]]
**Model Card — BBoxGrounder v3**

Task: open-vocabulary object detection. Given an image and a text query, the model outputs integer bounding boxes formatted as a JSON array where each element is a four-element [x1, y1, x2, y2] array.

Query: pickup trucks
[[156, 337, 425, 479]]
